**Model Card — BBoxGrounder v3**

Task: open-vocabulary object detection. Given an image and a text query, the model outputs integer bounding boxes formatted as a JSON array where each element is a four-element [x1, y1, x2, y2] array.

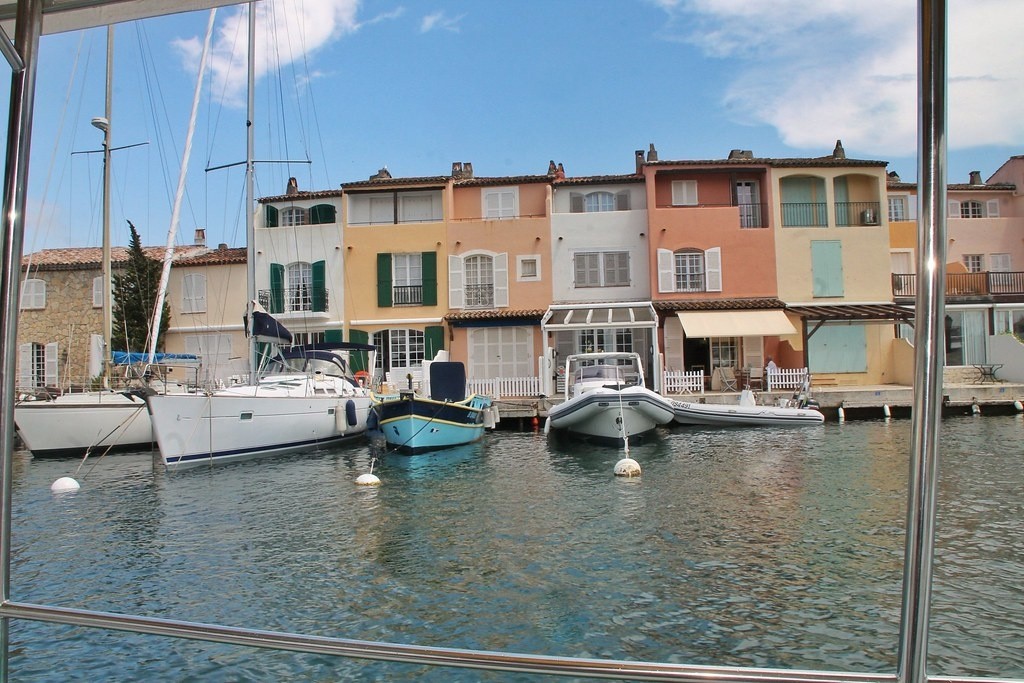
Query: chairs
[[670, 366, 693, 396], [749, 368, 764, 391], [715, 367, 738, 392]]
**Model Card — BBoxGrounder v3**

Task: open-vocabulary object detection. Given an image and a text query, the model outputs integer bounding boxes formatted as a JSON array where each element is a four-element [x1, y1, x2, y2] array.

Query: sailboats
[[15, 20, 206, 460], [138, 0, 379, 472]]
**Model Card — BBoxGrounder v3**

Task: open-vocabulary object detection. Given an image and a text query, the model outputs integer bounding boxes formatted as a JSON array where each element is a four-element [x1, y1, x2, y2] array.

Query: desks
[[968, 363, 1005, 384]]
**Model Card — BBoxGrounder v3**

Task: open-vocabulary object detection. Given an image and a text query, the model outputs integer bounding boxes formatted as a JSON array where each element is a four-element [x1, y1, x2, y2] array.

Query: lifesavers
[[354, 371, 370, 389]]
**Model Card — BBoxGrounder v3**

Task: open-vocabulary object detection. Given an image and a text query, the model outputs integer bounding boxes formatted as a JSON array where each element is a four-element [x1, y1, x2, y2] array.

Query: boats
[[370, 361, 492, 457], [665, 384, 825, 426], [544, 352, 675, 448]]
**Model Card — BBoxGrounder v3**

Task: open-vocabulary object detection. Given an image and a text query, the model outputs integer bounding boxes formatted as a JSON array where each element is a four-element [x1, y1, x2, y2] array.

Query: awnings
[[674, 308, 799, 339]]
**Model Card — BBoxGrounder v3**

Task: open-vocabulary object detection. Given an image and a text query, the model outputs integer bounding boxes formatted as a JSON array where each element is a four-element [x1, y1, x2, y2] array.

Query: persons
[[763, 356, 778, 390]]
